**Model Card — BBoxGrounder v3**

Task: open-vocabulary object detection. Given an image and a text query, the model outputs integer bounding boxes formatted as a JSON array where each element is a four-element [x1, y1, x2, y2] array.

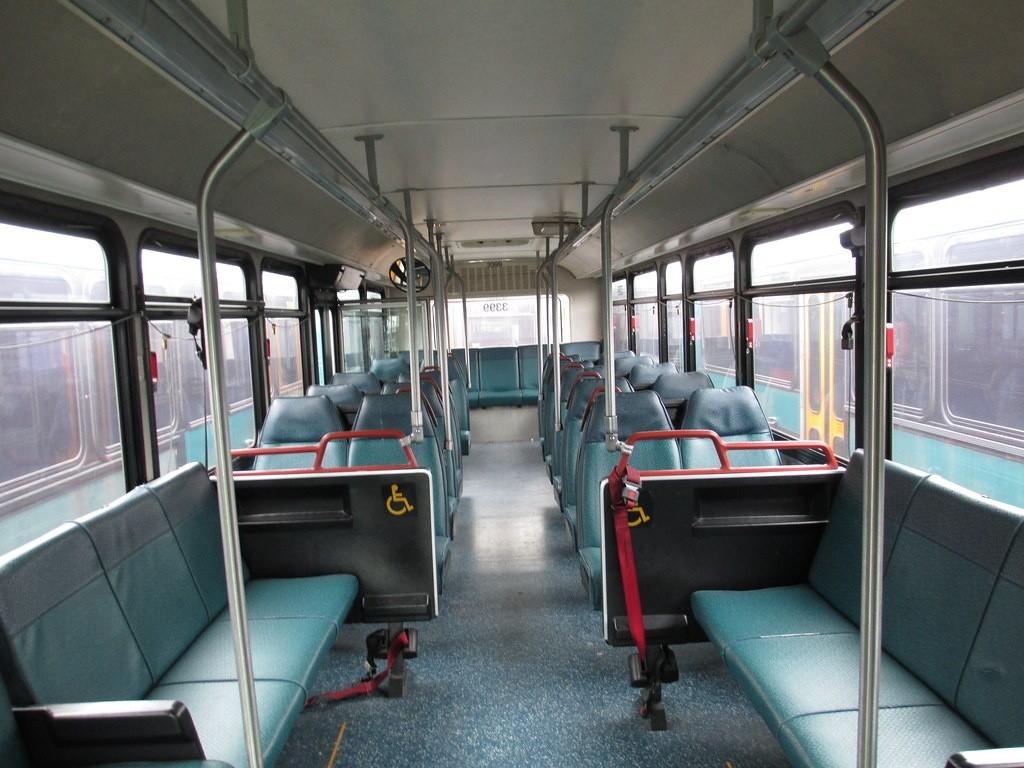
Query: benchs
[[397, 342, 599, 407], [0, 462, 360, 768], [691, 449, 1024, 768]]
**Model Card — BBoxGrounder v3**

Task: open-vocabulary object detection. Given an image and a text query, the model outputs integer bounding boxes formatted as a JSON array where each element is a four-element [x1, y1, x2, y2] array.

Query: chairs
[[420, 352, 470, 455], [381, 375, 457, 517], [545, 357, 595, 484], [561, 371, 635, 550], [552, 363, 606, 512], [369, 357, 407, 387], [680, 386, 783, 469], [305, 385, 362, 442], [347, 386, 451, 595], [330, 372, 381, 396], [653, 371, 716, 442], [578, 387, 683, 611], [599, 351, 635, 364], [253, 395, 349, 468], [629, 362, 679, 392], [398, 367, 463, 503], [614, 356, 655, 380], [539, 352, 584, 462]]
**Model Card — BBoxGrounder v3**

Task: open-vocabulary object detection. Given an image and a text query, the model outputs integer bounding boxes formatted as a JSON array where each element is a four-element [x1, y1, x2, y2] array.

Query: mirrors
[[391, 258, 429, 292]]
